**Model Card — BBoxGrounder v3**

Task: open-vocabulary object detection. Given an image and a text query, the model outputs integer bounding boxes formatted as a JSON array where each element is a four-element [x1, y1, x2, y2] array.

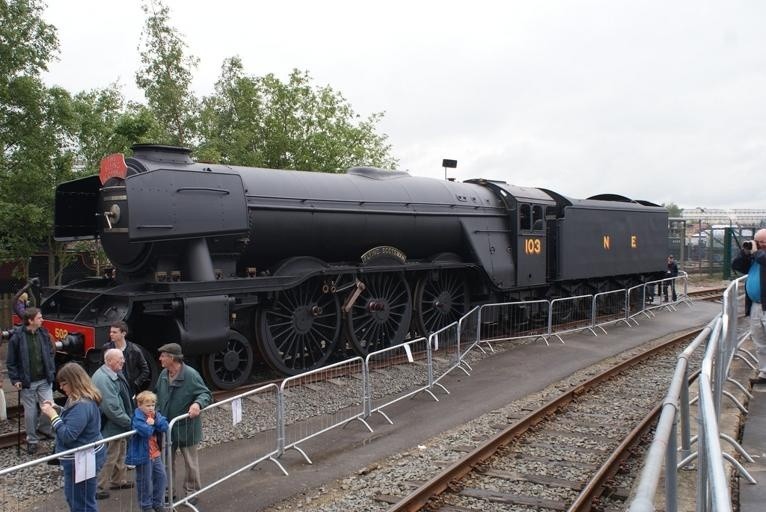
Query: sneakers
[[27, 428, 57, 454], [96, 464, 198, 512], [749, 376, 766, 384]]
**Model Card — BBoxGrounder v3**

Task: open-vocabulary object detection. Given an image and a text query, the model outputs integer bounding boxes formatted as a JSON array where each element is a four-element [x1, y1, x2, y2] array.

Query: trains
[[10, 143, 667, 391]]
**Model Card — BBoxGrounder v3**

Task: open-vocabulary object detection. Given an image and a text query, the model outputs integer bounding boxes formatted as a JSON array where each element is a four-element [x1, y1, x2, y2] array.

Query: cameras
[[740, 240, 759, 250]]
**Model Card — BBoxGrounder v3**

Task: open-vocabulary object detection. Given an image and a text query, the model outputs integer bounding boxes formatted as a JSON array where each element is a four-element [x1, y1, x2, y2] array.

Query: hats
[[157, 342, 184, 359]]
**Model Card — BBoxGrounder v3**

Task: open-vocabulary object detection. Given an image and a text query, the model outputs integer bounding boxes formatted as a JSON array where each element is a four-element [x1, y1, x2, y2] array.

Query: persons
[[6, 307, 57, 454], [40, 363, 107, 512], [152, 343, 213, 507], [98, 320, 151, 400], [125, 390, 171, 512], [662, 254, 678, 303], [658, 284, 661, 296], [731, 228, 766, 384], [91, 348, 136, 500], [14, 292, 28, 319]]
